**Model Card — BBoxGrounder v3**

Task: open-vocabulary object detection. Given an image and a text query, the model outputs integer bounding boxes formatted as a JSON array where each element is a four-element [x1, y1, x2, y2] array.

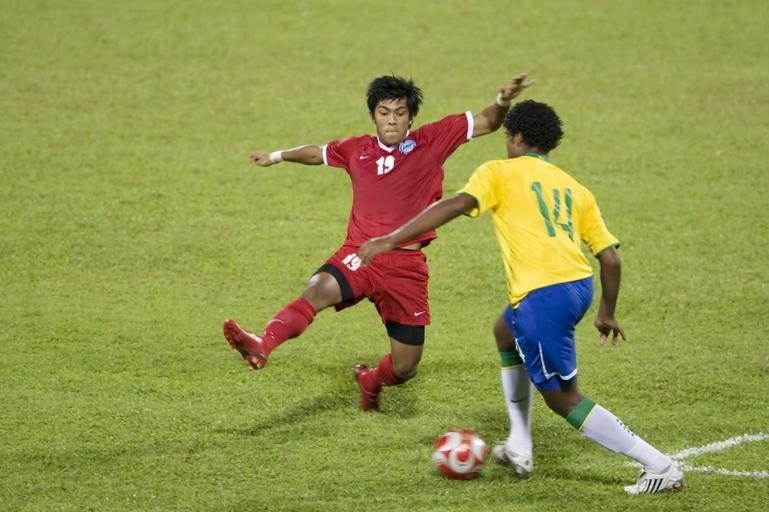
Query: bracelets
[[268, 149, 285, 166], [495, 92, 512, 108]]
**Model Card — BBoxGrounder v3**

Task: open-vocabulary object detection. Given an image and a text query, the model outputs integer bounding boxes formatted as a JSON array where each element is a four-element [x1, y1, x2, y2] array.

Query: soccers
[[430, 429, 489, 481]]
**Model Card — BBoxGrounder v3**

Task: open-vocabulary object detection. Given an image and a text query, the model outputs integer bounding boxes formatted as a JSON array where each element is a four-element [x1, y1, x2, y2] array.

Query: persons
[[354, 95, 688, 501], [222, 70, 537, 417]]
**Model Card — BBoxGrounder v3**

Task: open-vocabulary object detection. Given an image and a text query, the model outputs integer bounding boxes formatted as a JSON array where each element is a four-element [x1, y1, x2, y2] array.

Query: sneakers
[[621, 462, 688, 497], [352, 361, 381, 415], [222, 315, 268, 371], [490, 442, 536, 482]]
